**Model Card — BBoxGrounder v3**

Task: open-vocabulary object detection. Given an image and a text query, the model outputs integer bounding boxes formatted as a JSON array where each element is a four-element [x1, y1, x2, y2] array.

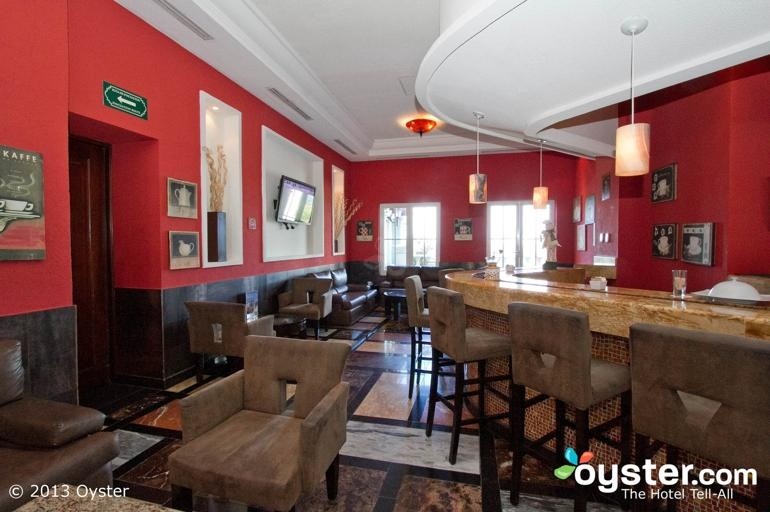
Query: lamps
[[531, 140, 549, 210], [469, 110, 488, 204], [612, 16, 652, 177], [400, 117, 439, 139]]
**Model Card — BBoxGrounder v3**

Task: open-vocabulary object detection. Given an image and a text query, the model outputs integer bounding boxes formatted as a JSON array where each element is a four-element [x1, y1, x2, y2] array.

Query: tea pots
[[174, 184, 193, 208], [178, 239, 195, 256]]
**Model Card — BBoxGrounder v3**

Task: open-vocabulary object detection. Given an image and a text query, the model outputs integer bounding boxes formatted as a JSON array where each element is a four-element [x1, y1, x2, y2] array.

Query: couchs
[[376, 265, 463, 307], [303, 265, 378, 327]]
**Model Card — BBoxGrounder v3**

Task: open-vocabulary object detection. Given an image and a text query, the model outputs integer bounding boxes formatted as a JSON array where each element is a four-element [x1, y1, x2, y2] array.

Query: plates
[[697, 289, 770, 304], [0, 212, 41, 218]]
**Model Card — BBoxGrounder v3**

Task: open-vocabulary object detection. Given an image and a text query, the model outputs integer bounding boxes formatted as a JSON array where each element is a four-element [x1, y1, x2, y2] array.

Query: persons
[[540, 219, 563, 262]]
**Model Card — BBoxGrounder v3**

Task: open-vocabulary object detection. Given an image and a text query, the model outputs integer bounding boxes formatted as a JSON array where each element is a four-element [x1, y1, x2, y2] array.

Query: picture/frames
[[166, 176, 199, 220], [453, 217, 474, 242], [652, 222, 679, 260], [650, 161, 678, 204], [680, 221, 714, 268], [355, 219, 374, 242], [169, 230, 201, 271], [570, 170, 611, 252]]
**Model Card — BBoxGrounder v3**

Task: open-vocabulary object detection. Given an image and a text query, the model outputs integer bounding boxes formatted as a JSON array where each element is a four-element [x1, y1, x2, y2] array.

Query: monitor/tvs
[[275, 175, 316, 226]]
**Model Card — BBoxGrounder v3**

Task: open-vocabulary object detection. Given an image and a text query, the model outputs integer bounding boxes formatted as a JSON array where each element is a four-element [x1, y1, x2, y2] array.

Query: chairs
[[1, 336, 122, 512], [182, 300, 276, 382], [279, 277, 333, 340], [425, 286, 513, 465], [505, 300, 632, 511], [403, 275, 459, 400], [626, 321, 770, 511], [164, 331, 354, 511], [437, 266, 464, 287]]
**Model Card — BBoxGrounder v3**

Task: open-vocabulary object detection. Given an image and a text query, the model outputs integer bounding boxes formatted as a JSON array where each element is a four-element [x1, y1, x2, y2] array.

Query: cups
[[3, 199, 34, 211], [672, 269, 687, 298]]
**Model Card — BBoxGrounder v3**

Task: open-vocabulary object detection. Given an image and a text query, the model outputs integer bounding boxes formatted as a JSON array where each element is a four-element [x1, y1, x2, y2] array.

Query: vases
[[334, 240, 339, 253], [208, 212, 227, 262]]
